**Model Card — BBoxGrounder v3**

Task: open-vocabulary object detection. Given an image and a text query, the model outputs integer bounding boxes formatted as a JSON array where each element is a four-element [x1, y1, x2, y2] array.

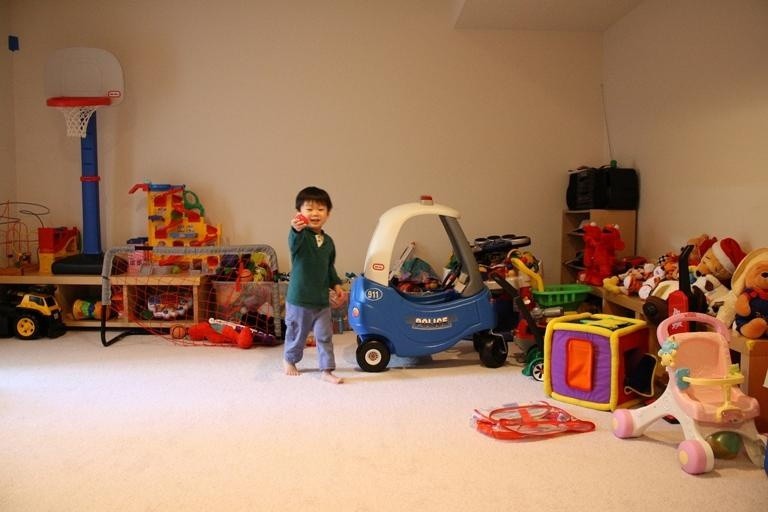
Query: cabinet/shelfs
[[557, 205, 636, 300], [603, 290, 767, 435], [2, 270, 214, 334]]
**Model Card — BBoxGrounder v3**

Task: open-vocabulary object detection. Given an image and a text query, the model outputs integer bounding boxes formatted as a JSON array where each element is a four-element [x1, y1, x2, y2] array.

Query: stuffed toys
[[580, 222, 768, 341]]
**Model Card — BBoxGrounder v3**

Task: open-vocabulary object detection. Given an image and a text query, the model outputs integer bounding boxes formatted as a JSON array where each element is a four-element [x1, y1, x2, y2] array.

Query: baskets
[[531, 284, 594, 306]]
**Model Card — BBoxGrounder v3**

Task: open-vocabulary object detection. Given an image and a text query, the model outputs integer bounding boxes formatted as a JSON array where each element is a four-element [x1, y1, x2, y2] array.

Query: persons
[[283, 186, 347, 383]]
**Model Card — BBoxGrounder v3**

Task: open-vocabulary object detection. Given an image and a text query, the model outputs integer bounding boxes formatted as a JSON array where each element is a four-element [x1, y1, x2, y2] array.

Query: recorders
[[566, 167, 639, 210]]
